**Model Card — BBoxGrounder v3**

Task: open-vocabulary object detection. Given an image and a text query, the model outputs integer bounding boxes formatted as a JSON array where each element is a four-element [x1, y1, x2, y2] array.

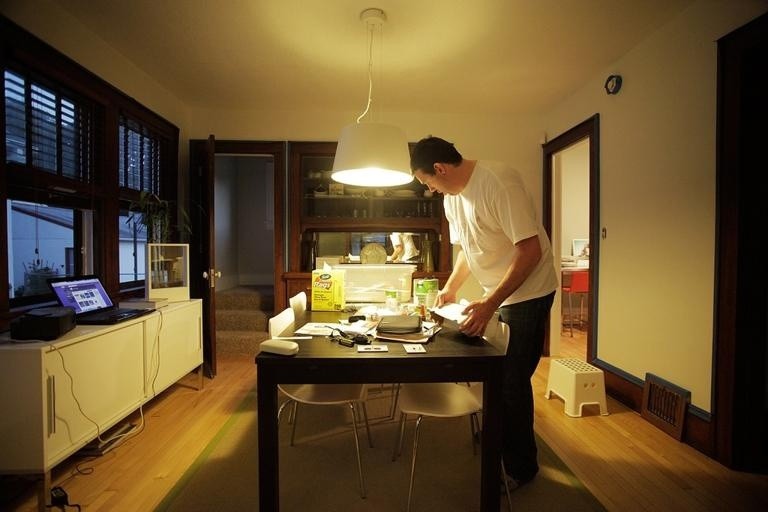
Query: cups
[[385, 289, 399, 311]]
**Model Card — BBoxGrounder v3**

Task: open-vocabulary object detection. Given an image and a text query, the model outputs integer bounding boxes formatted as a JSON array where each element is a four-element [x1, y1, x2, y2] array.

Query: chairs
[[562, 271, 589, 337]]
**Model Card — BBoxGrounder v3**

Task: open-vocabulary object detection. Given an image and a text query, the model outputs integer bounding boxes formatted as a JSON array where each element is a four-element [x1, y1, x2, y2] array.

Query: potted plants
[[125, 190, 192, 286]]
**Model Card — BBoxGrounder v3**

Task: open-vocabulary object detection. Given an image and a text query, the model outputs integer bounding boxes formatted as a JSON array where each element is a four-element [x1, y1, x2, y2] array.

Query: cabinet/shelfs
[[281, 142, 453, 311]]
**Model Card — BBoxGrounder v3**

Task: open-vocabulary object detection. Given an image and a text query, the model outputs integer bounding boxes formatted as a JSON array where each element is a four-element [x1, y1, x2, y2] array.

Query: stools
[[545, 357, 610, 417]]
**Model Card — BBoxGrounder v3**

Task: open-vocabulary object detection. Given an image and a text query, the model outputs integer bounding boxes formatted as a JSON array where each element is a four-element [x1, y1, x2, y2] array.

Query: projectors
[[11, 305, 78, 340]]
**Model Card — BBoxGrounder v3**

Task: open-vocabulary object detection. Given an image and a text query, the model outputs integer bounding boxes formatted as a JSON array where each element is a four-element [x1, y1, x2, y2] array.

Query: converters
[[51, 486, 69, 505]]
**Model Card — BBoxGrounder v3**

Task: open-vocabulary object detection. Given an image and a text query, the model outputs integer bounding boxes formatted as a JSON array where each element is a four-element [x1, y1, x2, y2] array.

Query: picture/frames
[[572, 239, 589, 269]]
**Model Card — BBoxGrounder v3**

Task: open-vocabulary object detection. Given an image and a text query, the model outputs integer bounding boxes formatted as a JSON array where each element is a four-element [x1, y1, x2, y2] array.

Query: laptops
[[46, 274, 157, 325]]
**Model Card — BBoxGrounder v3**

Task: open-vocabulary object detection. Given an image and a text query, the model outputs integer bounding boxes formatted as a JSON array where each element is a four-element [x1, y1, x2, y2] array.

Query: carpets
[[154, 382, 608, 511]]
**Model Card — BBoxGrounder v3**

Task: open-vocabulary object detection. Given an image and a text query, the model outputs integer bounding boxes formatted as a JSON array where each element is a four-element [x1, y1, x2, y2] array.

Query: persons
[[408, 135, 557, 496], [387, 233, 420, 264]]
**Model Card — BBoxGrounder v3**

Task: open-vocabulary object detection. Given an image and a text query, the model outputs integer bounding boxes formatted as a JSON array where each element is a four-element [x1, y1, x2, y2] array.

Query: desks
[[255, 311, 508, 512]]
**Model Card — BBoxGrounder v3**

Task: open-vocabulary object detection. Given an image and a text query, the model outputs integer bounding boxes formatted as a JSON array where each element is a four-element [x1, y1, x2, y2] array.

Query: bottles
[[414, 281, 428, 309]]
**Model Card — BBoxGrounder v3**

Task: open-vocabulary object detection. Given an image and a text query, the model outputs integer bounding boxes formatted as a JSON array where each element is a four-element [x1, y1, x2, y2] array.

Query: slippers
[[500, 467, 541, 495]]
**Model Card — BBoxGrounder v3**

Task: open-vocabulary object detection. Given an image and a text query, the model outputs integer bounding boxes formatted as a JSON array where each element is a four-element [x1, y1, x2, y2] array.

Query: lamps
[[331, 8, 414, 187]]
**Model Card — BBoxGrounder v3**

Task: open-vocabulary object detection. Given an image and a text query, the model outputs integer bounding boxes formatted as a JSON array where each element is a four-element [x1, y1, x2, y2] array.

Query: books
[[292, 316, 443, 345]]
